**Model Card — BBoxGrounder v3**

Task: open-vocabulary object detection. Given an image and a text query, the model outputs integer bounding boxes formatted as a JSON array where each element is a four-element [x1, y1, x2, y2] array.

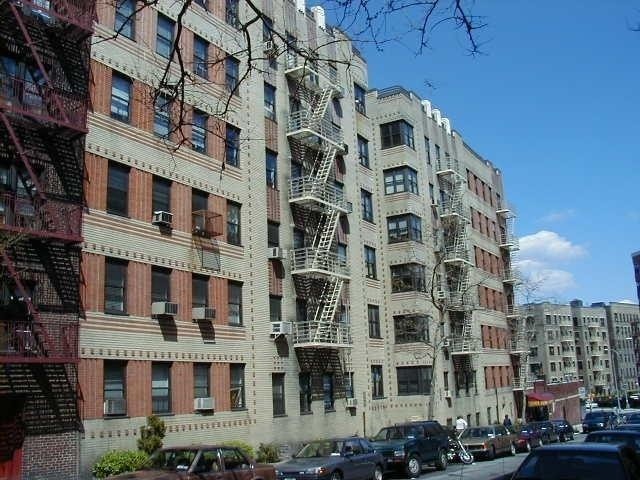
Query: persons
[[455, 415, 468, 430], [503, 414, 512, 426]]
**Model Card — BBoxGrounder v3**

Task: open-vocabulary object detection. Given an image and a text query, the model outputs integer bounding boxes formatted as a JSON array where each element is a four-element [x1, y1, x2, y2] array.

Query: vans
[[582, 411, 617, 431]]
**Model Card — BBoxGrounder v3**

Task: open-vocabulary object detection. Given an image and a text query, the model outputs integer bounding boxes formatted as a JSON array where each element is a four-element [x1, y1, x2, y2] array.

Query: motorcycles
[[448, 429, 472, 464]]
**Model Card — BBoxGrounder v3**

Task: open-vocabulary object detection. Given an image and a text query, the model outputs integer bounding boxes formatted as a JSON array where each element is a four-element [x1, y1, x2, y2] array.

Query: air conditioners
[[268, 246, 283, 259], [151, 301, 216, 321], [152, 211, 174, 224], [270, 321, 292, 335], [193, 397, 216, 410], [348, 398, 359, 407]]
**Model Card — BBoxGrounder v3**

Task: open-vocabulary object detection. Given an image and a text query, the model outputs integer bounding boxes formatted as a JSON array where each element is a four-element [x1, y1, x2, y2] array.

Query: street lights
[[604, 347, 630, 409]]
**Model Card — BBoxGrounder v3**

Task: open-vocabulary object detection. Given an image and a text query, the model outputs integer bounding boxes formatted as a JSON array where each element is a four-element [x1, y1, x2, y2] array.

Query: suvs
[[372, 420, 449, 477]]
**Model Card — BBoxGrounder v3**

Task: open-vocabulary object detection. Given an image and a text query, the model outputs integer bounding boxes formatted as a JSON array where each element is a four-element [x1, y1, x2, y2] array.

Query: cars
[[276, 437, 386, 479], [494, 431, 639, 479], [458, 424, 519, 460], [619, 412, 640, 430], [511, 419, 575, 452], [106, 444, 277, 480]]
